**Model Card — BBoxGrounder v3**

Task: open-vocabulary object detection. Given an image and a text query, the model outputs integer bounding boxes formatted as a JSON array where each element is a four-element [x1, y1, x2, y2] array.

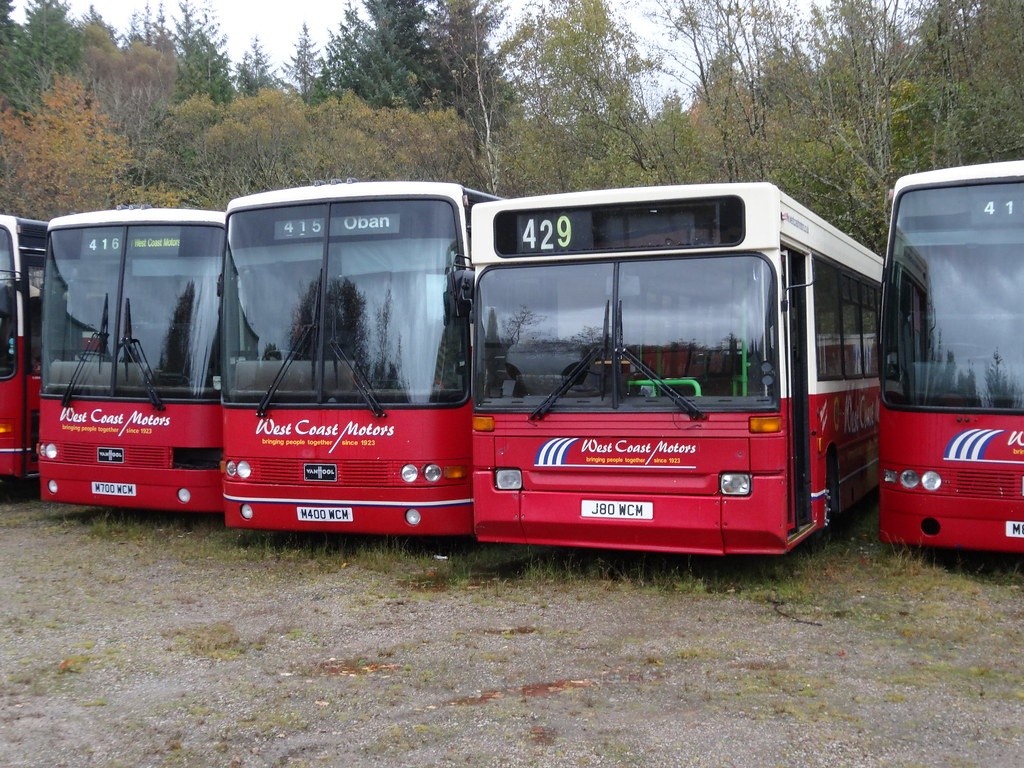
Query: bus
[[873, 161, 1024, 558], [445, 181, 883, 555], [1, 214, 54, 479], [37, 209, 227, 518], [217, 181, 732, 540]]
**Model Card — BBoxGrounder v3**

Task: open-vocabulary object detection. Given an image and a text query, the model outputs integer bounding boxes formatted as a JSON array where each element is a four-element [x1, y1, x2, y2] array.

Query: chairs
[[642, 343, 855, 386]]
